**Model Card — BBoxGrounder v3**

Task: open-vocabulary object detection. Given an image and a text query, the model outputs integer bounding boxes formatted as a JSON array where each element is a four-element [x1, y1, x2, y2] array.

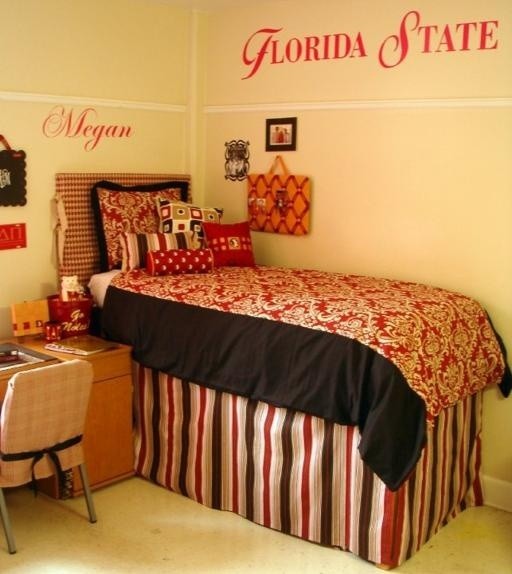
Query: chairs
[[0, 358, 101, 556]]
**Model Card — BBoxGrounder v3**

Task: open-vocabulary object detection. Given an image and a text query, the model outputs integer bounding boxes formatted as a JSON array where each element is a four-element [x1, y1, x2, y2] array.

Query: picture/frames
[[264, 116, 299, 153]]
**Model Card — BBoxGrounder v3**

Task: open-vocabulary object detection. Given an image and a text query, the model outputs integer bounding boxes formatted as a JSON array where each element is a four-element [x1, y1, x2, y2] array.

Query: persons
[[277, 130, 284, 143], [282, 126, 291, 143], [271, 125, 280, 143]]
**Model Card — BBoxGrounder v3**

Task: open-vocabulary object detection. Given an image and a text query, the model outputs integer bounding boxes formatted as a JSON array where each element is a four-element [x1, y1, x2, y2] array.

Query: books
[[45, 333, 119, 357]]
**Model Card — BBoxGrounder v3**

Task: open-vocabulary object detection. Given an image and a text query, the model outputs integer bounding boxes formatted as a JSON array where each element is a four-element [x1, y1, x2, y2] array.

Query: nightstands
[[0, 326, 140, 501]]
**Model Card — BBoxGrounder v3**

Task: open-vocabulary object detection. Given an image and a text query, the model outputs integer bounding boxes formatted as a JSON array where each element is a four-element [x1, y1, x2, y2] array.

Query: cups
[[44, 320, 62, 343]]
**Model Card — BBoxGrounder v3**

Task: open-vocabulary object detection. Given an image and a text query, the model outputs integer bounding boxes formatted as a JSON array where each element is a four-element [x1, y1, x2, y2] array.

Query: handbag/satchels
[[246, 156, 311, 238]]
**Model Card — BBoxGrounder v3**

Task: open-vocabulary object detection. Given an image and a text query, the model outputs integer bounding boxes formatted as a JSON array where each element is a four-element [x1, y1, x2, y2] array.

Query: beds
[[50, 173, 512, 568]]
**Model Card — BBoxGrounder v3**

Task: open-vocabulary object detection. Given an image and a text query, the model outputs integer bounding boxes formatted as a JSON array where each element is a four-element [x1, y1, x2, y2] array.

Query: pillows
[[90, 181, 256, 278]]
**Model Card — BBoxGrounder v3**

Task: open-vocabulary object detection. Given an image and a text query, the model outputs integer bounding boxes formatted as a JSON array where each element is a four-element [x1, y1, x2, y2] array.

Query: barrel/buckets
[[46, 290, 94, 337]]
